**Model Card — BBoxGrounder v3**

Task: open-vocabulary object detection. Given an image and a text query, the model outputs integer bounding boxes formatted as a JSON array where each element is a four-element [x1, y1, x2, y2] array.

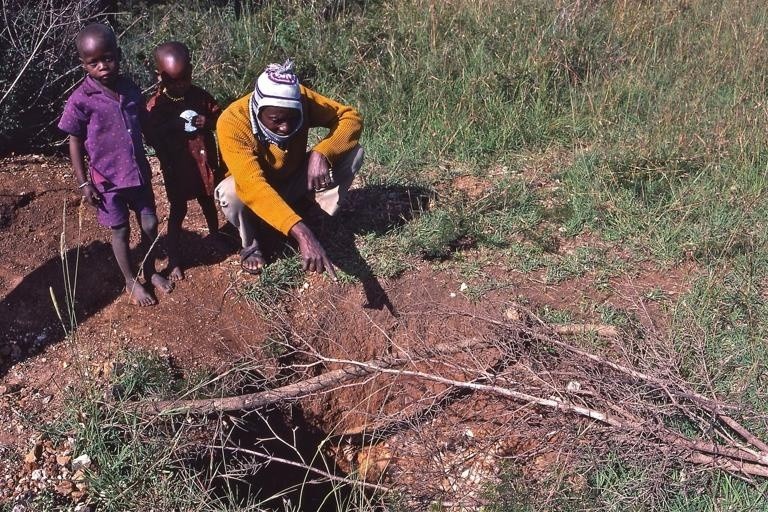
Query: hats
[[248, 59, 303, 144]]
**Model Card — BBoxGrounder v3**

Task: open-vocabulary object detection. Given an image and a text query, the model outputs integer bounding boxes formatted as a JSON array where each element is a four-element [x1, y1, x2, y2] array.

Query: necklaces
[[165, 92, 185, 103]]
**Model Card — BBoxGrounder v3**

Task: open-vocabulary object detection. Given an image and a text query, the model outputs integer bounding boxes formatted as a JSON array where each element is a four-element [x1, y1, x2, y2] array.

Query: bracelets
[[78, 181, 92, 190]]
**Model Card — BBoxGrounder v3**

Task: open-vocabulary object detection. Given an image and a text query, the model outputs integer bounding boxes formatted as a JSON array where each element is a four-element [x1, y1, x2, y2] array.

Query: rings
[[321, 183, 326, 186]]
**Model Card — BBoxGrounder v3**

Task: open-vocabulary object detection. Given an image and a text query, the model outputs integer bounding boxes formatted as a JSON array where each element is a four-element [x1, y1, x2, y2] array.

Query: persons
[[141, 43, 224, 281], [213, 64, 365, 283], [56, 22, 174, 306]]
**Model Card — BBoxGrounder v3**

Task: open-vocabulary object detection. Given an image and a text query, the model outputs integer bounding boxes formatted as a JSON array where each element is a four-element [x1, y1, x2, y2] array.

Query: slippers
[[296, 205, 323, 226], [240, 238, 269, 274]]
